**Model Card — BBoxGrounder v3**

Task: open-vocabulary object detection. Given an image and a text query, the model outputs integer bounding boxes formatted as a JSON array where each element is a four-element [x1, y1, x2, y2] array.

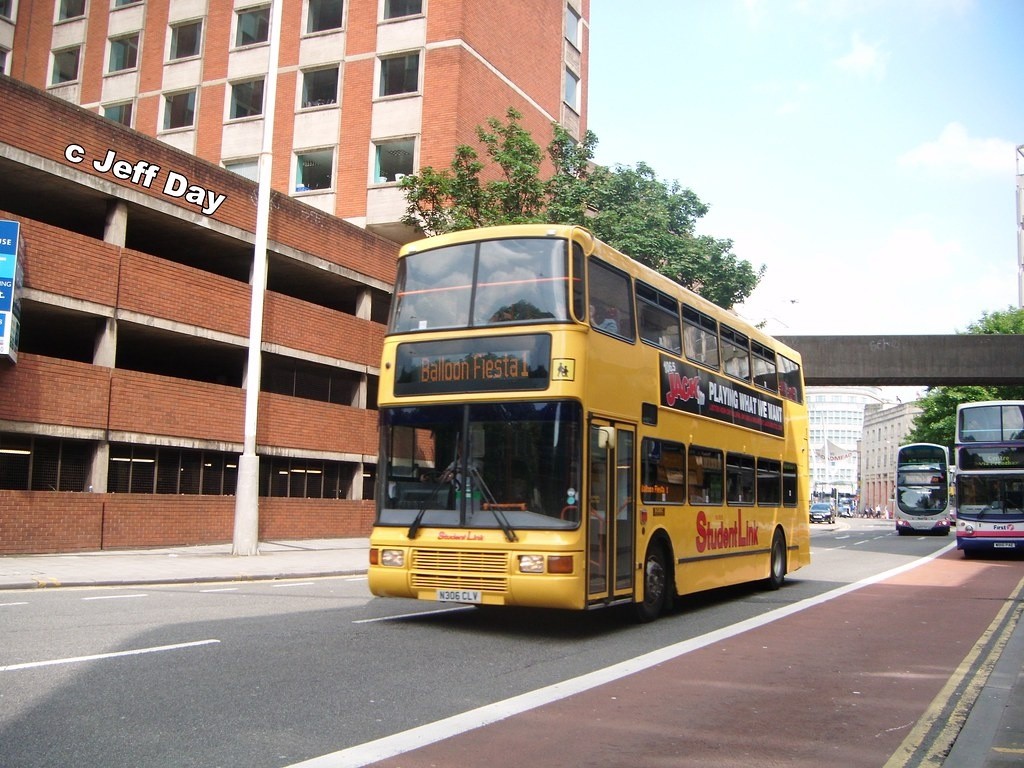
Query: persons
[[874, 504, 882, 518], [861, 503, 869, 518], [582, 296, 619, 334], [419, 437, 484, 497], [883, 504, 890, 519], [868, 504, 875, 518], [988, 480, 1001, 502]]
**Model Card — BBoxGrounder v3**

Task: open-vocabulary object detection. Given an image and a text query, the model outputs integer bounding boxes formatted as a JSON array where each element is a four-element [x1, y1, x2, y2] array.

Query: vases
[[395, 172, 404, 182]]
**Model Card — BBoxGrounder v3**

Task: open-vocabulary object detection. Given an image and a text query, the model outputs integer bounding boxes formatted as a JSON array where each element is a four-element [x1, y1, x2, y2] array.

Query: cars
[[809, 503, 836, 524]]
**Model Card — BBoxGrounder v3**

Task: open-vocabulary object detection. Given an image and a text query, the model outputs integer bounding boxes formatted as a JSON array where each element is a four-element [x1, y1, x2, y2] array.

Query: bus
[[891, 442, 956, 535], [955, 395, 1024, 564], [366, 220, 816, 619]]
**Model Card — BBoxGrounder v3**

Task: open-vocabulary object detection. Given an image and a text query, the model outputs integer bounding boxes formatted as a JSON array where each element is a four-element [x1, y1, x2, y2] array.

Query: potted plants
[[378, 170, 387, 183]]
